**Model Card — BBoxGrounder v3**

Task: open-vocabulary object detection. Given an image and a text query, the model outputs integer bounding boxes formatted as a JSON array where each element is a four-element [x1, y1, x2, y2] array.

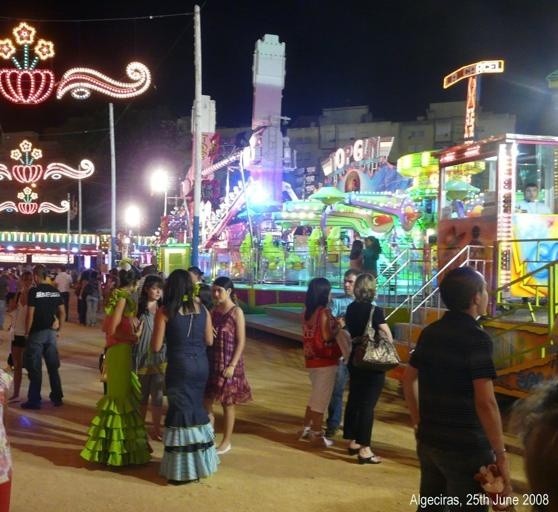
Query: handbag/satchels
[[354, 306, 401, 372]]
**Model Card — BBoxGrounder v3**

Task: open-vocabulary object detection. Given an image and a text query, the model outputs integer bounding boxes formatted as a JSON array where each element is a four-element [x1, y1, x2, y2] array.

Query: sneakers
[[51, 399, 63, 407], [312, 436, 334, 447], [20, 402, 40, 410], [298, 431, 311, 443], [325, 427, 343, 440]]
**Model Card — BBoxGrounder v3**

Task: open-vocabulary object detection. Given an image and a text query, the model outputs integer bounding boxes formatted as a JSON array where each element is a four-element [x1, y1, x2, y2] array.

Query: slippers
[[358, 455, 382, 465], [349, 446, 359, 455]]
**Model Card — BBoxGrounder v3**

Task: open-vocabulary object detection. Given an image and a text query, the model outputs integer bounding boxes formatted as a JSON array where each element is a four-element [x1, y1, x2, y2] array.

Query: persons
[[351, 179, 360, 191], [515, 183, 552, 214], [463, 225, 484, 272], [507, 379, 557, 512], [401, 266, 513, 512], [298, 236, 393, 464]]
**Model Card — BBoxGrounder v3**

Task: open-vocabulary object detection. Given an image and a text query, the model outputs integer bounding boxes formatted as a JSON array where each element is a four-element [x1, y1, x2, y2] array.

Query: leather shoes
[[216, 443, 231, 455], [6, 397, 20, 403]]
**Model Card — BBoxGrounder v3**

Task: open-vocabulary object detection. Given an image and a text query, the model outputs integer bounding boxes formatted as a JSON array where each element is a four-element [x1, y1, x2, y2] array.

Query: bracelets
[[493, 448, 506, 455]]
